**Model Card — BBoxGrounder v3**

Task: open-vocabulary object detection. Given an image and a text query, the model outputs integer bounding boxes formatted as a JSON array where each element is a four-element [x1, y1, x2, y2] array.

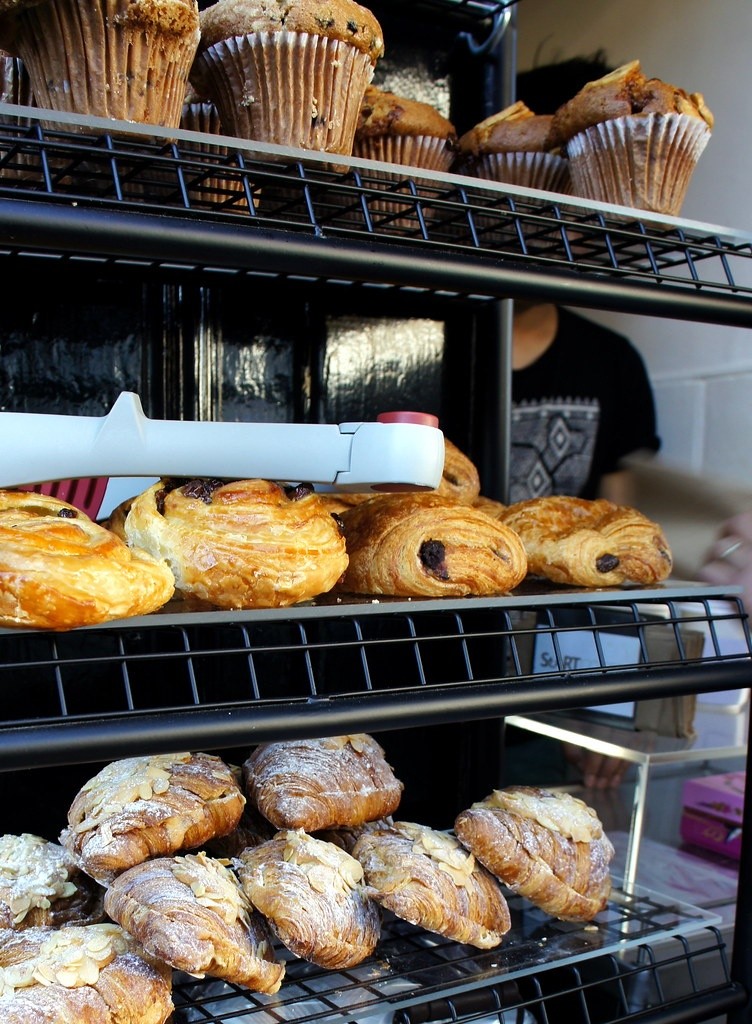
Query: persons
[[563, 716, 641, 790], [508, 289, 665, 520], [693, 501, 751, 626]]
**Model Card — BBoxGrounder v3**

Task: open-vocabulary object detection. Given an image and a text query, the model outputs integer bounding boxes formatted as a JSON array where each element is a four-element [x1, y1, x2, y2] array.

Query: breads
[[67, 750, 245, 886], [0, 490, 174, 628], [0, 834, 97, 929], [125, 474, 349, 608], [234, 830, 381, 970], [333, 495, 528, 597], [496, 497, 671, 587], [102, 854, 286, 995], [0, 922, 175, 1024], [455, 784, 614, 920], [331, 439, 480, 503], [244, 734, 401, 831], [355, 822, 510, 947]]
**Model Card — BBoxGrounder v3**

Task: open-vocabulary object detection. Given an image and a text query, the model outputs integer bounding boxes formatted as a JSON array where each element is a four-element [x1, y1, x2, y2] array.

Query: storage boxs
[[679, 770, 746, 861]]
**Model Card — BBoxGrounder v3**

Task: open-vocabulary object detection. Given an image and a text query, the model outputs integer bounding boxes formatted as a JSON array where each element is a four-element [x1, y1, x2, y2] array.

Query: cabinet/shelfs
[[0, 98, 752, 1024]]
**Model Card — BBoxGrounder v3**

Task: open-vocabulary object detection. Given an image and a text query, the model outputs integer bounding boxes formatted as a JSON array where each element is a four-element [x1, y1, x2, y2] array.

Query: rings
[[719, 540, 742, 559]]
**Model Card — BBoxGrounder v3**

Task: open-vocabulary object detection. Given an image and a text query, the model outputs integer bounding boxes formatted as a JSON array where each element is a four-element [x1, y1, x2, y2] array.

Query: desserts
[[0, 0, 714, 235]]
